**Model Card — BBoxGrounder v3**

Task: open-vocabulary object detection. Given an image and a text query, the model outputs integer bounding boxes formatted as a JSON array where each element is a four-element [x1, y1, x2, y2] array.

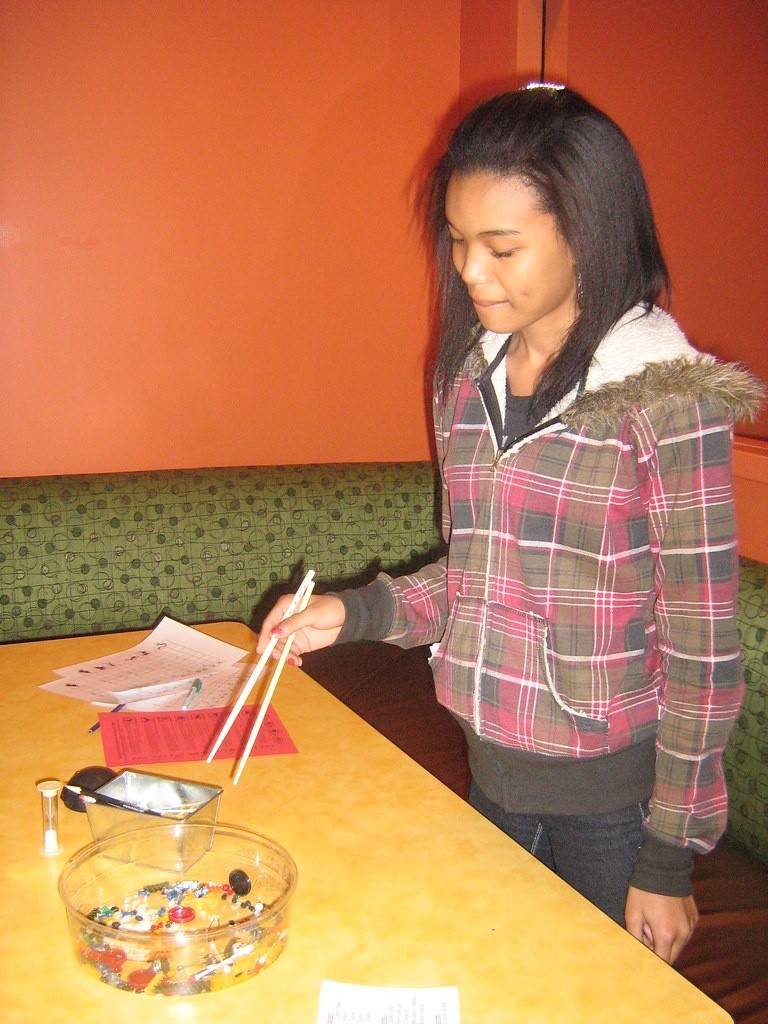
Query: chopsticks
[[205, 569, 316, 787]]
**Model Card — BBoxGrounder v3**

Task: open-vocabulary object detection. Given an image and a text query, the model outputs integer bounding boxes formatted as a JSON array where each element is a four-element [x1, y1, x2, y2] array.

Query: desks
[[0, 621, 735, 1024]]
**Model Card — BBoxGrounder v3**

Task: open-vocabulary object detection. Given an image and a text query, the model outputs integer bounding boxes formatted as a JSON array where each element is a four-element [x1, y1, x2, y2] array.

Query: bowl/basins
[[57, 822, 300, 1001]]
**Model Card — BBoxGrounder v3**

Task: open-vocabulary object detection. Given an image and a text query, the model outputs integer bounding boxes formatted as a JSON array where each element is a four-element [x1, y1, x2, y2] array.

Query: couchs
[[0, 460, 768, 1024]]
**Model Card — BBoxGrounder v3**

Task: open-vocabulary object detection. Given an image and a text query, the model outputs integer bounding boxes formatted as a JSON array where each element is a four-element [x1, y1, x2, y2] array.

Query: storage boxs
[[82, 767, 224, 875]]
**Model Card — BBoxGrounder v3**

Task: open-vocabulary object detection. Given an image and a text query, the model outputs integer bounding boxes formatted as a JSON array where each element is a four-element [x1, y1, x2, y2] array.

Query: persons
[[256, 84, 762, 965]]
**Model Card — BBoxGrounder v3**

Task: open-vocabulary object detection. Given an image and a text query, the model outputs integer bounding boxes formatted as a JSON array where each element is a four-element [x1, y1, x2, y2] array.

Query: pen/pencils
[[181, 678, 203, 711], [89, 704, 125, 733], [64, 785, 163, 818]]
